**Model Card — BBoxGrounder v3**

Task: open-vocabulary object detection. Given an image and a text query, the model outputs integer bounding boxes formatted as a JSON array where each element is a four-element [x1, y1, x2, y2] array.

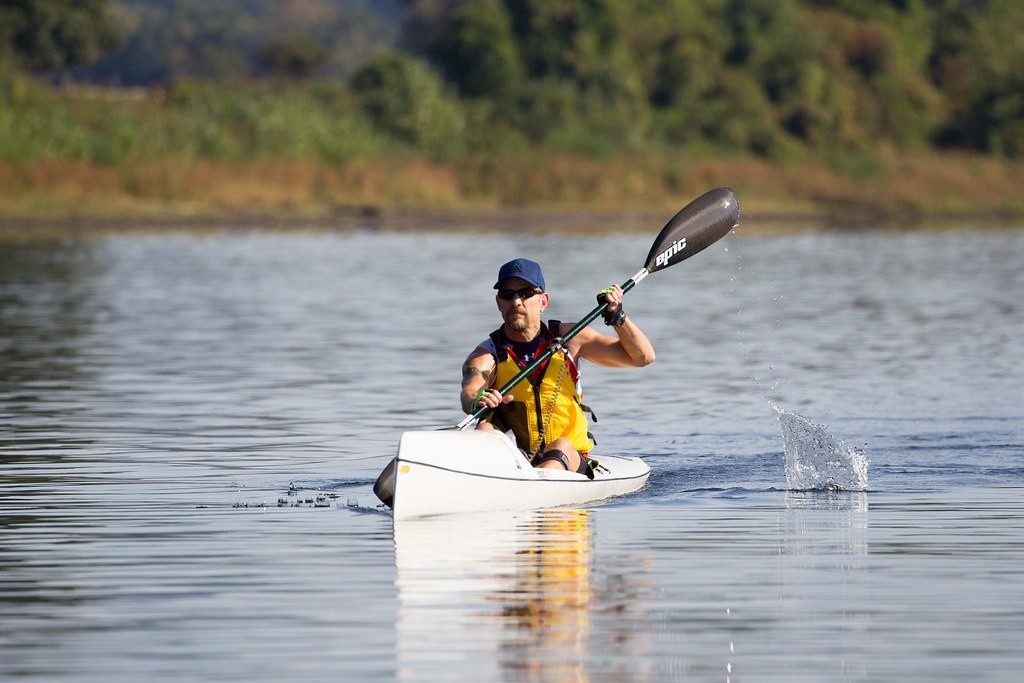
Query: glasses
[[498, 287, 543, 300]]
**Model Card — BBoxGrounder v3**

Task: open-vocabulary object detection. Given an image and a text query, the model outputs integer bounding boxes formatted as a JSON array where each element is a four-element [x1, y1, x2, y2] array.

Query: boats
[[370, 427, 653, 518]]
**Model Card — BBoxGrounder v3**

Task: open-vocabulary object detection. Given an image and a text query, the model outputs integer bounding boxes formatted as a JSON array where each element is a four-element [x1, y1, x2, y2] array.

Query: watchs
[[610, 310, 626, 326]]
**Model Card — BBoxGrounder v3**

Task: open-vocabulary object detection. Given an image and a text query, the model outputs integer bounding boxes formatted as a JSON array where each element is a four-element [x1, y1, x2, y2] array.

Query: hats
[[494, 258, 545, 292]]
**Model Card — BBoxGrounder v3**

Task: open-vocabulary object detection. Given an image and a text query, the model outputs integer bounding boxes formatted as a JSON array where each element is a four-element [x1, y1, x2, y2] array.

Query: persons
[[461, 259, 655, 474]]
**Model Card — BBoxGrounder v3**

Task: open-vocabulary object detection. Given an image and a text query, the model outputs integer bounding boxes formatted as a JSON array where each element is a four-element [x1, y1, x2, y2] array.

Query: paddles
[[372, 185, 741, 514]]
